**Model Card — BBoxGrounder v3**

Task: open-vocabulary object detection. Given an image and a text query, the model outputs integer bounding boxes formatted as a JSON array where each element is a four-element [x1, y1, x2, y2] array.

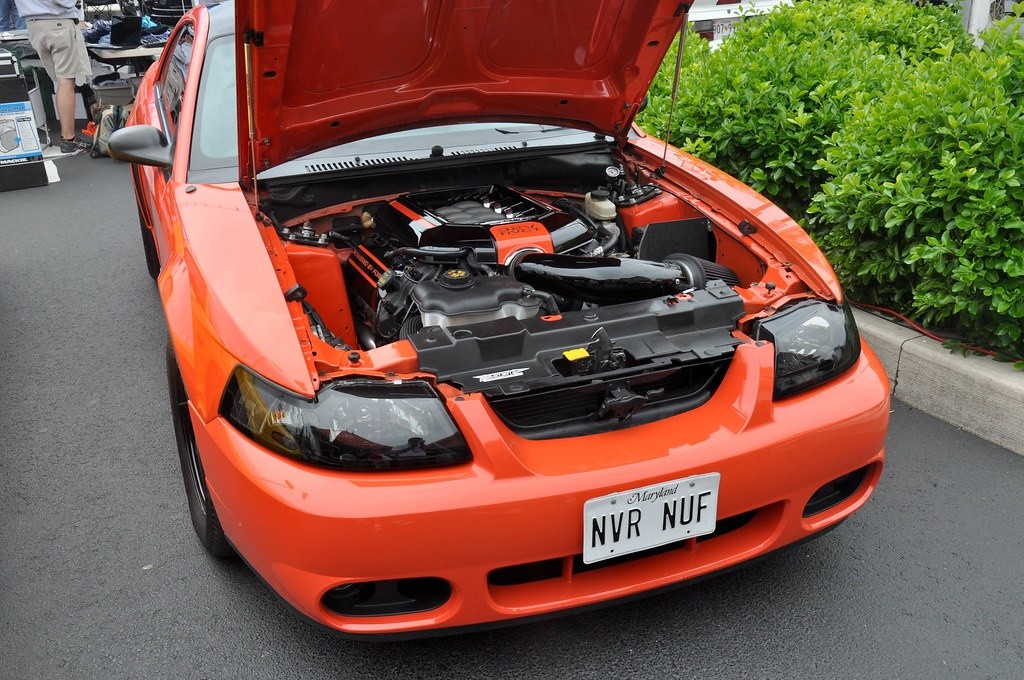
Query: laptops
[[86, 16, 142, 49]]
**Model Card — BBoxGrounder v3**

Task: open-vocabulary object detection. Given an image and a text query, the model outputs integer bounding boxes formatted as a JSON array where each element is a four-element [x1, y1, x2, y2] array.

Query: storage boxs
[[91, 79, 135, 106]]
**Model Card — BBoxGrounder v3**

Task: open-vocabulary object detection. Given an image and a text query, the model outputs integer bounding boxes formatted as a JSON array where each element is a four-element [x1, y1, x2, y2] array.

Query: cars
[[108, 0, 892, 632]]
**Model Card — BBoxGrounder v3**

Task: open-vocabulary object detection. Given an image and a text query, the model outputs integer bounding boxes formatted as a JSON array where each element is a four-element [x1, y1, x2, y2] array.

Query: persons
[[14, 0, 93, 154]]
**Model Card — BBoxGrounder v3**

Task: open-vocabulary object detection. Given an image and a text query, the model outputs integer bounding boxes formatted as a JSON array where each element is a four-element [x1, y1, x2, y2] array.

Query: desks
[[88, 45, 165, 77]]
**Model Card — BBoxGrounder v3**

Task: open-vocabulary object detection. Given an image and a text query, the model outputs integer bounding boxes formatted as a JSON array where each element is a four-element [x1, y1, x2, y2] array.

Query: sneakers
[[61, 136, 91, 155]]
